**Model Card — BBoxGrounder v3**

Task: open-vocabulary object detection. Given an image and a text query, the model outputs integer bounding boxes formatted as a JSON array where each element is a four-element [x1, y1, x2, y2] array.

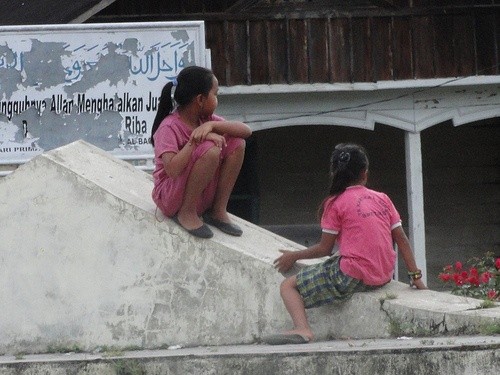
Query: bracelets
[[408, 270, 422, 286]]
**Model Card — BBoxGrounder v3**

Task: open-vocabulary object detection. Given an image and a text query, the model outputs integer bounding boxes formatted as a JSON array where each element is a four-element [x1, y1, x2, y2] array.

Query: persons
[[264, 142, 427, 345], [151, 66, 252, 239]]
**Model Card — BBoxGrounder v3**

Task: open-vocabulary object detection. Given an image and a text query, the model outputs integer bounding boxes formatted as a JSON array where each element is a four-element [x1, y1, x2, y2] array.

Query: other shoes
[[173, 213, 213, 238], [203, 210, 242, 236]]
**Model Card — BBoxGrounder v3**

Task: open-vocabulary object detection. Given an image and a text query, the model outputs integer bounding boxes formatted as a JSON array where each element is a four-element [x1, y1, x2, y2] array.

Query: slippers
[[260, 331, 309, 345]]
